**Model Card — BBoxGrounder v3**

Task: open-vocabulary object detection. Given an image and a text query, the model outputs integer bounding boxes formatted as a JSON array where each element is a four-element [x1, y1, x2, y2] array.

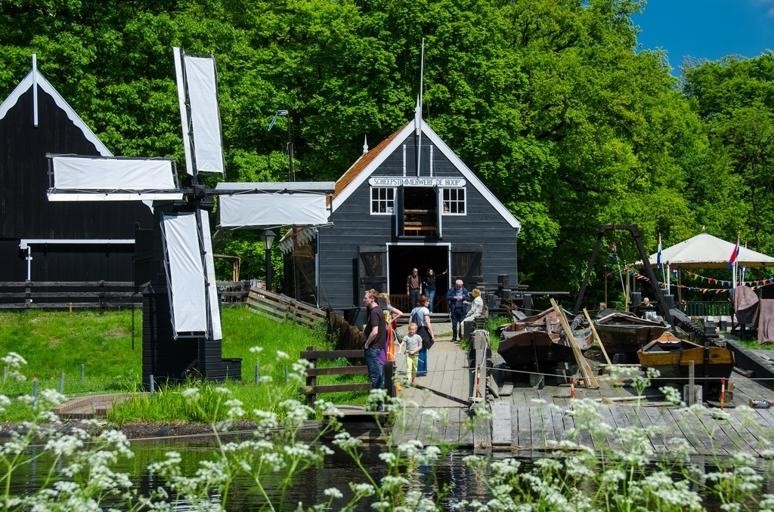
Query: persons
[[446, 279, 484, 342], [597, 303, 607, 313], [397, 323, 422, 388], [363, 289, 403, 412], [409, 296, 435, 377], [643, 297, 653, 307], [423, 267, 448, 313], [407, 268, 423, 313]]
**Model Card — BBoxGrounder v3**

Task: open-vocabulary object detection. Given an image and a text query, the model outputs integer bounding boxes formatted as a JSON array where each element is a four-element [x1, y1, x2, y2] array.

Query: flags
[[727, 239, 739, 269], [656, 240, 662, 271]]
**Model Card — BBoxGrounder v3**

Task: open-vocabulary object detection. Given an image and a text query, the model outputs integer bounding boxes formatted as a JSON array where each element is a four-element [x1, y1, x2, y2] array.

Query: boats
[[496, 301, 739, 402]]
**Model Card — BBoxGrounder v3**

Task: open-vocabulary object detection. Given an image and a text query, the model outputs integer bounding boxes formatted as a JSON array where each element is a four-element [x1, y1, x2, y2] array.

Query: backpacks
[[410, 309, 421, 329]]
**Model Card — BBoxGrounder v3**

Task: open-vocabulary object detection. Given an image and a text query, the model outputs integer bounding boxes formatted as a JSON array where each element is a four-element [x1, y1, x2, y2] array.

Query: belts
[[410, 288, 420, 290]]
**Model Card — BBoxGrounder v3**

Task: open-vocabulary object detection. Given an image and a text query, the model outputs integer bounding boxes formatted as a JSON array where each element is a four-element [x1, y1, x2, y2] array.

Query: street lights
[[256, 228, 279, 301]]
